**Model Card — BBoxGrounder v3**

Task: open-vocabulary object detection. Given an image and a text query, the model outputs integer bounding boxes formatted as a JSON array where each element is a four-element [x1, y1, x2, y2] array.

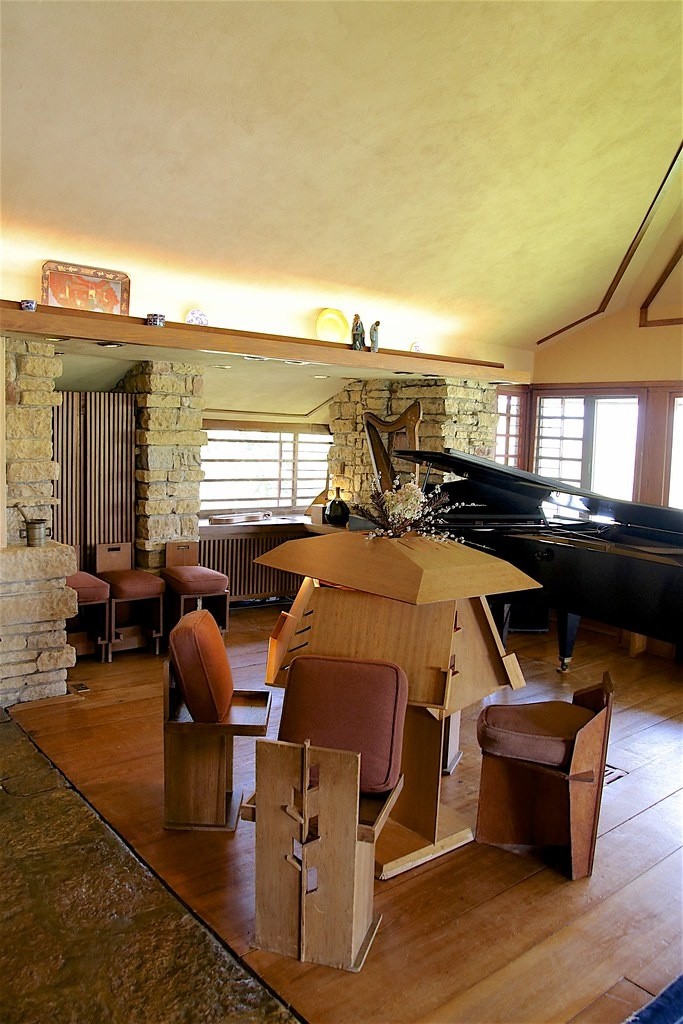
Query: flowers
[[352, 474, 486, 543]]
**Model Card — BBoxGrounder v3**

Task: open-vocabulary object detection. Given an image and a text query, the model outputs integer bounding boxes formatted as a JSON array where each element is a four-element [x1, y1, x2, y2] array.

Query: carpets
[[621, 973, 682, 1024]]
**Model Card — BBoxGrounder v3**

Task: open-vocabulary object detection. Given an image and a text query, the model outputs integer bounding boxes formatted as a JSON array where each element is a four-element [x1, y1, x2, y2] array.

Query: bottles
[[325, 487, 351, 527]]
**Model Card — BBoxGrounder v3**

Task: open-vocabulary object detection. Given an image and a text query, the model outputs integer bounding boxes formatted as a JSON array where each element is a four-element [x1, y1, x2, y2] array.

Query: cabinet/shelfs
[[198, 519, 349, 603]]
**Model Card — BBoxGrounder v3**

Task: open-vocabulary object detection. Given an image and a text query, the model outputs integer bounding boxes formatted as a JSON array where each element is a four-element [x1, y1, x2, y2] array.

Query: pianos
[[496, 483, 683, 674], [392, 449, 554, 636]]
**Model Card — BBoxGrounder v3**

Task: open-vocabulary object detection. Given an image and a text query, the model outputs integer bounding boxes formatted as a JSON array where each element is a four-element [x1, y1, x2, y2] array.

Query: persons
[[351, 314, 381, 353]]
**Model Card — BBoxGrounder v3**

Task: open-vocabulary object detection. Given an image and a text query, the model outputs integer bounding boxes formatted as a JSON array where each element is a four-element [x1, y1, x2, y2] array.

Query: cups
[[20, 519, 51, 547], [21, 300, 37, 310], [147, 314, 165, 327]]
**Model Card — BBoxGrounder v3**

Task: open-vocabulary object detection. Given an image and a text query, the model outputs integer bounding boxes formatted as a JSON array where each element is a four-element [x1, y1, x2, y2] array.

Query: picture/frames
[[40, 261, 130, 316]]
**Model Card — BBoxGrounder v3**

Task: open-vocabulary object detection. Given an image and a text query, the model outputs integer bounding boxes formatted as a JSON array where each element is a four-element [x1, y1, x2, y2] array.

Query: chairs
[[161, 611, 271, 835], [473, 670, 615, 879], [236, 653, 405, 974], [66, 572, 110, 664], [96, 541, 165, 666], [164, 542, 230, 632]]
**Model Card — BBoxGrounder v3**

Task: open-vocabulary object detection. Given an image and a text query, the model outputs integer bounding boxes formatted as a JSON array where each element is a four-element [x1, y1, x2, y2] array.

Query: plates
[[185, 310, 208, 326], [315, 308, 350, 342]]
